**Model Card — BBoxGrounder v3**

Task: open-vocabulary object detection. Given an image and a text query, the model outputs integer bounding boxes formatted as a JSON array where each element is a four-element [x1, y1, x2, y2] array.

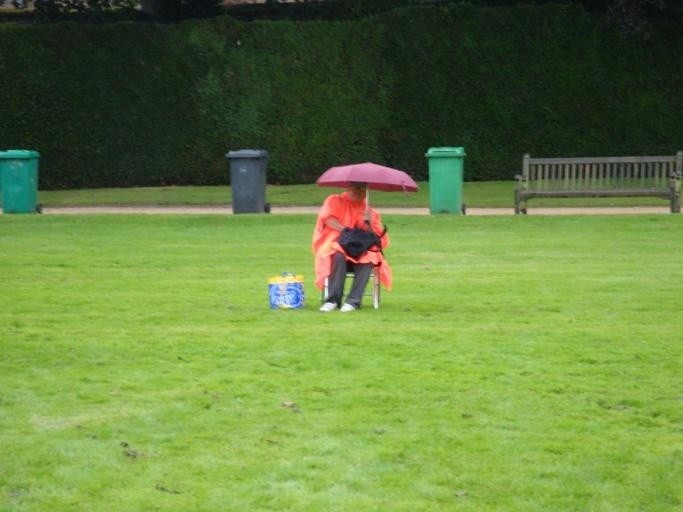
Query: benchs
[[514, 151, 683, 214]]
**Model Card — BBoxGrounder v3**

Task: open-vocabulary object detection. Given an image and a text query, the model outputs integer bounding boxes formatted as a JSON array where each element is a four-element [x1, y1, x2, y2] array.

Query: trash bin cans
[[224, 149, 271, 213], [424, 146, 468, 216], [0, 149, 43, 214]]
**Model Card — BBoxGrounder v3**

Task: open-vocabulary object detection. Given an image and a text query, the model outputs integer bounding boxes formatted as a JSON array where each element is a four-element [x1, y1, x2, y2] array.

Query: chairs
[[323, 272, 380, 310]]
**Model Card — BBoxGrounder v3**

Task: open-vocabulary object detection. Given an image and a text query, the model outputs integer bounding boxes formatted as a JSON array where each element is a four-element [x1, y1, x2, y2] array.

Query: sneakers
[[319, 300, 339, 312], [339, 302, 357, 312]]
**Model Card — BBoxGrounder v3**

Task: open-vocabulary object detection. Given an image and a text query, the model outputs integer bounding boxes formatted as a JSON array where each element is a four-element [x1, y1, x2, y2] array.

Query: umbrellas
[[317, 162, 418, 195]]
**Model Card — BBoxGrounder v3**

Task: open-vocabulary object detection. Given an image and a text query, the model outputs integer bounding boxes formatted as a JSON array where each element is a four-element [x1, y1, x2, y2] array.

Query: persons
[[311, 181, 389, 311]]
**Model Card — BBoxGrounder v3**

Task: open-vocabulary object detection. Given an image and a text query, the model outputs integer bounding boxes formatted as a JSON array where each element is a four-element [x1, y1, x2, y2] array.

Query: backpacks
[[337, 224, 380, 260]]
